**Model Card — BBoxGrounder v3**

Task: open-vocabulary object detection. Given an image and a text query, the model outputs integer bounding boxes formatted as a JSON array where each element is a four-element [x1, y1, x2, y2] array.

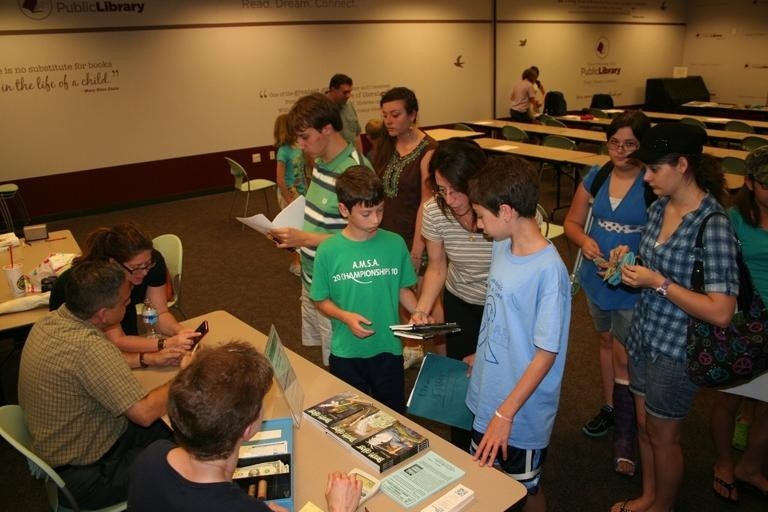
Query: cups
[[3, 263, 27, 300]]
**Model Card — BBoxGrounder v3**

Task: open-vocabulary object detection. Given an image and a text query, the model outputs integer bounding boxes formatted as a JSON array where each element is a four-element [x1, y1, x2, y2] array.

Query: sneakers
[[401, 346, 425, 370], [583, 405, 616, 437]]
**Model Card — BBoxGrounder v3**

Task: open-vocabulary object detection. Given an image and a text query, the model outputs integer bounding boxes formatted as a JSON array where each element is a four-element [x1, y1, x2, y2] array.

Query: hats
[[626, 122, 707, 162], [744, 145, 768, 184]]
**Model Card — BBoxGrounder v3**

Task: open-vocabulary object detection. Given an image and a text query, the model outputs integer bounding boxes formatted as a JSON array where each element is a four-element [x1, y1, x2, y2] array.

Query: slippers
[[732, 415, 752, 451], [713, 464, 739, 505], [608, 498, 636, 512]]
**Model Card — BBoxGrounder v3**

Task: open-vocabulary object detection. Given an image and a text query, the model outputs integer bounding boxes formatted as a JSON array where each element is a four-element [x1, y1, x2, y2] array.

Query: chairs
[[136, 233, 190, 321], [0, 179, 31, 233], [419, 88, 768, 262], [0, 404, 127, 511], [224, 154, 276, 231]]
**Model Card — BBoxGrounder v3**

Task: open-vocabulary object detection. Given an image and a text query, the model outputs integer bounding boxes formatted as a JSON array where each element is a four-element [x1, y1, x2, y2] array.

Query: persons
[[274, 73, 494, 415], [457, 152, 572, 511], [124, 335, 363, 511], [71, 219, 203, 350], [561, 108, 661, 479], [606, 117, 740, 510], [17, 255, 169, 510], [509, 67, 545, 125], [709, 143, 766, 512]]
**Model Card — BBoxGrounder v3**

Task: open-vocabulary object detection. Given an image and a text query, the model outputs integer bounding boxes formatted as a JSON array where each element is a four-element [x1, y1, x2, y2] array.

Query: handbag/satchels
[[685, 212, 768, 392]]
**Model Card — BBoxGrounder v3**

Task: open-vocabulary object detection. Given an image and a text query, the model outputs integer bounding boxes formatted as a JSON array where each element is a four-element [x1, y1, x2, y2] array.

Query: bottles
[[141, 298, 162, 339]]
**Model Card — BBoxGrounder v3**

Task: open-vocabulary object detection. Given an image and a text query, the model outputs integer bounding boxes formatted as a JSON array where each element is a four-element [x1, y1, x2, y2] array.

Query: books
[[303, 387, 467, 510], [405, 352, 475, 431], [0, 232, 21, 254]]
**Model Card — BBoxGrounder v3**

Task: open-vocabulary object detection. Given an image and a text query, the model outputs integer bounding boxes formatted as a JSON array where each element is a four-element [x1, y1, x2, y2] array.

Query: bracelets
[[139, 351, 148, 368], [493, 409, 514, 425], [157, 337, 166, 350]]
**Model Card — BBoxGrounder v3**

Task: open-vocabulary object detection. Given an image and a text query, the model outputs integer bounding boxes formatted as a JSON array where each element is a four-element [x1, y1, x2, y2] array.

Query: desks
[[1, 230, 86, 333], [124, 310, 528, 511], [682, 101, 768, 113]]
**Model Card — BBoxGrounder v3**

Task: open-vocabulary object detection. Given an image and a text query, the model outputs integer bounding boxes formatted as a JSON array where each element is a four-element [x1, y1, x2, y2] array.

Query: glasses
[[431, 184, 457, 198], [119, 261, 157, 274], [605, 141, 640, 152]]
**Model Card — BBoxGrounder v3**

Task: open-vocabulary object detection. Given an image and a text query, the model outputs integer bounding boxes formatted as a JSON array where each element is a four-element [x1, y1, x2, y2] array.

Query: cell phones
[[188, 321, 209, 348]]
